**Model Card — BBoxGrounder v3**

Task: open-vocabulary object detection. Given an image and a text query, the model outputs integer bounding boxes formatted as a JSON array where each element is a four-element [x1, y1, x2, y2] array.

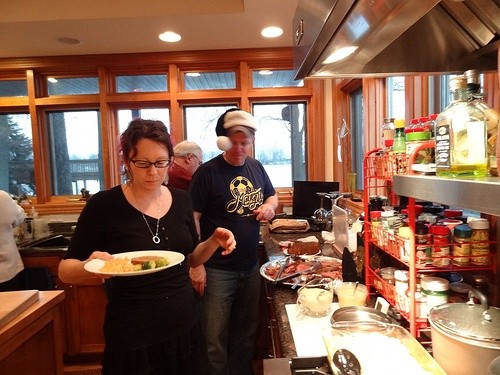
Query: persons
[[0, 190, 26, 291], [188, 108, 278, 375], [59, 120, 236, 375], [162, 140, 203, 192]]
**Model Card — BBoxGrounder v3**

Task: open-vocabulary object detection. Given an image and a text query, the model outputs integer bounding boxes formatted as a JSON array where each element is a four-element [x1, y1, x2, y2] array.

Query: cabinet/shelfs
[[21, 257, 109, 355], [362, 142, 500, 339]]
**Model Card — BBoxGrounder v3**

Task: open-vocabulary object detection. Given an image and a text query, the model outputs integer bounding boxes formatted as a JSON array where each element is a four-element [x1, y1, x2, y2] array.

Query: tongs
[[273, 257, 320, 285]]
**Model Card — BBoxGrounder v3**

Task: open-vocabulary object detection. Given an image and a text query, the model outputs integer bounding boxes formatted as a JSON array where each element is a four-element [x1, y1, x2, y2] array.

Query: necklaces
[[131, 184, 162, 243]]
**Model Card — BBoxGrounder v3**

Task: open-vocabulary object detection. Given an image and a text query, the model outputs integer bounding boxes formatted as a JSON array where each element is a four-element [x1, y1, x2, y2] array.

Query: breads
[[287, 241, 320, 255]]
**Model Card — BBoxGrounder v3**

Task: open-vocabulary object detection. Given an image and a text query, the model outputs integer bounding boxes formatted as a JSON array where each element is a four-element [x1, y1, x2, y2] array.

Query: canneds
[[370, 206, 490, 268], [24, 217, 34, 238], [379, 266, 477, 319], [405, 131, 432, 175]]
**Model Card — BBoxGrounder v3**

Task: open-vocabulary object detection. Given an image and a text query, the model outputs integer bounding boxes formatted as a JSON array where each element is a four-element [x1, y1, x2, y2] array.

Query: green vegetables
[[142, 259, 167, 269]]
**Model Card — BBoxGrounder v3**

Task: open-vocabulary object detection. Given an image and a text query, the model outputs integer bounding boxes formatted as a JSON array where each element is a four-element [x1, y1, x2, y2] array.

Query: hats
[[215, 108, 257, 151]]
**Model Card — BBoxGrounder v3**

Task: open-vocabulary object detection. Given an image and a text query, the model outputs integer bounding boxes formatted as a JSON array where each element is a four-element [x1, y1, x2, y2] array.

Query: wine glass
[[314, 191, 352, 231]]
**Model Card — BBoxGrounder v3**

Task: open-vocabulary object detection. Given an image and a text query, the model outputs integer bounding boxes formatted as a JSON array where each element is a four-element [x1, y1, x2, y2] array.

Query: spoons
[[332, 348, 361, 375]]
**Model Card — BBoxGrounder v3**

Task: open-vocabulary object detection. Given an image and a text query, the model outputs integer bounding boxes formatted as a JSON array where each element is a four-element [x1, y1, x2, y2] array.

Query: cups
[[297, 278, 334, 317], [335, 281, 368, 307]]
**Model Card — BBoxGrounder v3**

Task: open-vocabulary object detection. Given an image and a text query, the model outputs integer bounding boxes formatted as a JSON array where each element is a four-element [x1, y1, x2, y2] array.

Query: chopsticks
[[331, 306, 395, 334]]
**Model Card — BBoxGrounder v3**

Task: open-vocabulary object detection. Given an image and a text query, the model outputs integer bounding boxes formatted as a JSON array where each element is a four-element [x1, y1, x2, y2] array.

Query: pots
[[428, 288, 500, 375]]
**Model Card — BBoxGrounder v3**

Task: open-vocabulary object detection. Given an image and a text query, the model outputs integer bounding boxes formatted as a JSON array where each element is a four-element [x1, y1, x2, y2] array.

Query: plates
[[284, 248, 321, 255], [260, 255, 343, 287], [84, 250, 186, 275]]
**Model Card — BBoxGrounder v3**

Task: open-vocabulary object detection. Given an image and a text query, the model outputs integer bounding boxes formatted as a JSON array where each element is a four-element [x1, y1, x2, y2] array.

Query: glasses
[[192, 154, 202, 166], [129, 159, 171, 168]]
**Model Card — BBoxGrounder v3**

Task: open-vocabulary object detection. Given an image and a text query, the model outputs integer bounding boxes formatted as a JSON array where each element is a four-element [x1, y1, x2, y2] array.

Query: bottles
[[381, 69, 500, 181], [375, 268, 488, 324]]
